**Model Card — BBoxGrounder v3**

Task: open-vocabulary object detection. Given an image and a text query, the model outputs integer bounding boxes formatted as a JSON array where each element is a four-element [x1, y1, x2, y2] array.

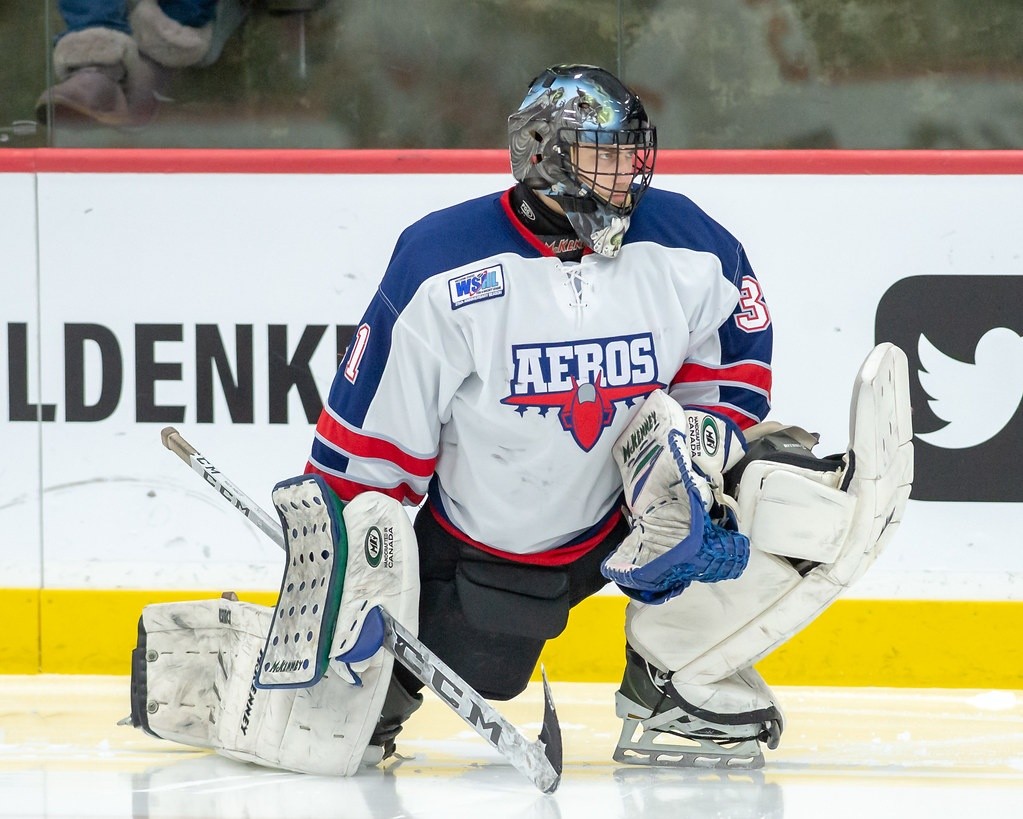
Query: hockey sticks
[[160, 423, 564, 797]]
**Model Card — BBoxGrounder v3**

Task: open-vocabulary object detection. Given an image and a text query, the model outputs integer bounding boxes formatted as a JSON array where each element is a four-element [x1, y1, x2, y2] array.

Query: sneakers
[[613, 641, 764, 774], [613, 767, 783, 819]]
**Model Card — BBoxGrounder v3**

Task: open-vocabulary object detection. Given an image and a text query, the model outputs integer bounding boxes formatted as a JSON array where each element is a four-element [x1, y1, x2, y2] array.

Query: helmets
[[507, 63, 656, 258]]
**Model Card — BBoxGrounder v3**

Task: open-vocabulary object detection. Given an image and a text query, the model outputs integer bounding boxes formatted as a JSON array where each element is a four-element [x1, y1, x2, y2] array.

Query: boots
[[36, 26, 160, 129], [128, 0, 214, 102]]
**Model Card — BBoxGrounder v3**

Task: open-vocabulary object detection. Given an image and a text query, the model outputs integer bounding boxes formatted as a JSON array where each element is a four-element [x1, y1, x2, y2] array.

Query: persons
[[37, 1, 218, 127], [130, 64, 915, 780]]
